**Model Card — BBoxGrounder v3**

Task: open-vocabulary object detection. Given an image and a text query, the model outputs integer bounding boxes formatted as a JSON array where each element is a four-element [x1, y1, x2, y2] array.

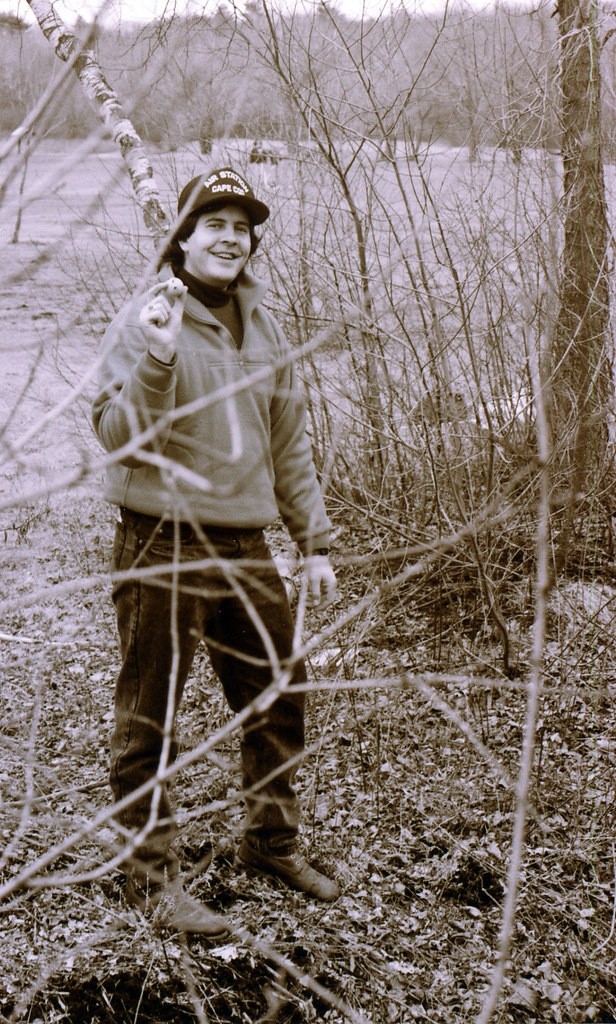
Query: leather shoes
[[127, 880, 228, 941], [233, 839, 340, 903]]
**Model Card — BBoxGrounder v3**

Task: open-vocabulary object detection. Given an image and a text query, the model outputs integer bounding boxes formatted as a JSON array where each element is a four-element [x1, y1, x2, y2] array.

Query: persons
[[91, 168, 340, 937]]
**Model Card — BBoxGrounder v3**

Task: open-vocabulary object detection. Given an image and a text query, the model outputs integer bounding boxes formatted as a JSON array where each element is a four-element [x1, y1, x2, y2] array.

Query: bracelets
[[304, 549, 328, 555]]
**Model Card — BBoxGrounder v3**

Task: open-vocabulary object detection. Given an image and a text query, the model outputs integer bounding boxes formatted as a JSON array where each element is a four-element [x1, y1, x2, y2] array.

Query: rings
[[148, 306, 155, 312]]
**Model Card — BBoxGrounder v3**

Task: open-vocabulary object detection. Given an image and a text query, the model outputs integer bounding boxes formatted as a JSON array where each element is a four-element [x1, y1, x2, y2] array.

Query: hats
[[177, 167, 270, 224]]
